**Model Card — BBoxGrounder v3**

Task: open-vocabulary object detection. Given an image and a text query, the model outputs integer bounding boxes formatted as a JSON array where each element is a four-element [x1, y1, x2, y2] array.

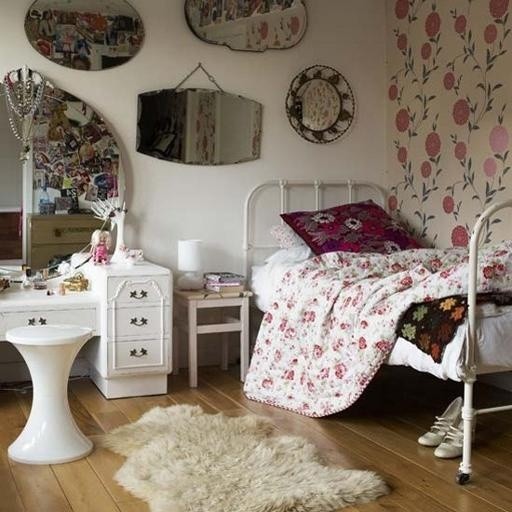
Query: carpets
[[102, 404, 393, 512]]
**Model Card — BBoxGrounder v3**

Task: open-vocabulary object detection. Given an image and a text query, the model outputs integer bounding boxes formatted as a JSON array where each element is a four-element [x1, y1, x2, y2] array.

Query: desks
[[0, 258, 176, 401]]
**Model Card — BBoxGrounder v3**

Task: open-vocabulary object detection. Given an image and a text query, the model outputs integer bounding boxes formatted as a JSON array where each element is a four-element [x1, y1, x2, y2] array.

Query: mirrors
[[0, 83, 132, 285]]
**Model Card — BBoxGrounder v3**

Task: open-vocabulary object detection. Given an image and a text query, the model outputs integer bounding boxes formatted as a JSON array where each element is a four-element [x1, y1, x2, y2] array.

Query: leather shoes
[[417, 397, 463, 446], [434, 408, 477, 458]]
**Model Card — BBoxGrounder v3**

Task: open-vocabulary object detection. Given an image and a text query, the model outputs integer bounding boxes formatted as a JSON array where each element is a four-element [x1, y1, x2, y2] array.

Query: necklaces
[[3, 64, 43, 162]]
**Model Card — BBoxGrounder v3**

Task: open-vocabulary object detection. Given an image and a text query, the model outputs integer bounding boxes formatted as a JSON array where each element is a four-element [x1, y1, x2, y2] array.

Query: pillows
[[272, 196, 427, 267]]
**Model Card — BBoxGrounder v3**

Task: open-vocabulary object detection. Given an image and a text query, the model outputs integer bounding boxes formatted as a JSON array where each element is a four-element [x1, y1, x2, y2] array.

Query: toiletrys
[[23, 266, 33, 289]]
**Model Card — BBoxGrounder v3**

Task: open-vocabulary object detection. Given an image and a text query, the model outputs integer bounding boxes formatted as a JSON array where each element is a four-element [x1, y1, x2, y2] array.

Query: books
[[204, 271, 244, 292]]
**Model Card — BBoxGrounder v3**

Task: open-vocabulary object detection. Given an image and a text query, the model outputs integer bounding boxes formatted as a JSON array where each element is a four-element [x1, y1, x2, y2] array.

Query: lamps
[[175, 239, 205, 289]]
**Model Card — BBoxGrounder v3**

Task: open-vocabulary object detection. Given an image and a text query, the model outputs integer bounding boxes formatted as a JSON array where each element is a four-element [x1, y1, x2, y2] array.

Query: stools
[[4, 327, 96, 468], [178, 283, 253, 389]]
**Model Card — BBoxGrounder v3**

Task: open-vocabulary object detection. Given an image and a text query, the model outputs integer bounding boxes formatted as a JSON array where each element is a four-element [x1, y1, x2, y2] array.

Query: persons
[[72, 55, 90, 70], [59, 12, 67, 24], [118, 32, 127, 45], [63, 32, 72, 57], [92, 13, 109, 43], [77, 39, 90, 60], [90, 229, 112, 265], [129, 29, 141, 50], [39, 10, 57, 42]]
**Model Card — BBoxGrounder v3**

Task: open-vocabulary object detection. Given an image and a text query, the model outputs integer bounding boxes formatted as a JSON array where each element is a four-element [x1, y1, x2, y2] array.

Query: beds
[[240, 177, 511, 485]]
